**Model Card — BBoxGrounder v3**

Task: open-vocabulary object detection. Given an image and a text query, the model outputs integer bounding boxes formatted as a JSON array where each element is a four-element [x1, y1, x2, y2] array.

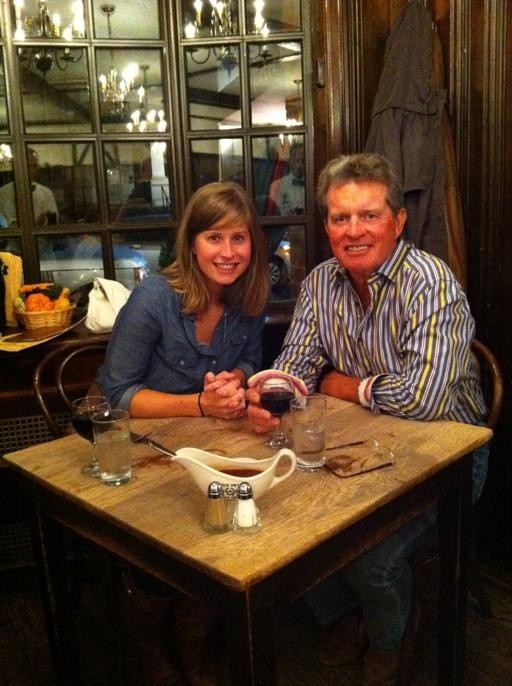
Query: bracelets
[[196, 392, 208, 421]]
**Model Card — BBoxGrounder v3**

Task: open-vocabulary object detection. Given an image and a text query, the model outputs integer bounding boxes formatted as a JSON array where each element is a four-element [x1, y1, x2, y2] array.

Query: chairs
[[35, 335, 186, 686], [398, 339, 503, 686]]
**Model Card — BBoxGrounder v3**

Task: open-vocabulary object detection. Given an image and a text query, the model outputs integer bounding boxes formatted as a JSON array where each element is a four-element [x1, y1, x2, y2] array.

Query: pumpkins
[[25, 293, 53, 312]]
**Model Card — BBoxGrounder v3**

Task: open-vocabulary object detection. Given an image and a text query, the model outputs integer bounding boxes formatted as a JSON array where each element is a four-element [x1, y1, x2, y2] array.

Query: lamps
[[10, 0, 87, 76], [127, 64, 167, 133], [182, 0, 280, 77], [87, 4, 137, 123], [278, 80, 303, 149]]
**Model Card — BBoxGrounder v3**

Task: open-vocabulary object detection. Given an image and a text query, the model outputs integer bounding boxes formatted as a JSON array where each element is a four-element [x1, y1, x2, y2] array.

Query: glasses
[[324, 436, 394, 478]]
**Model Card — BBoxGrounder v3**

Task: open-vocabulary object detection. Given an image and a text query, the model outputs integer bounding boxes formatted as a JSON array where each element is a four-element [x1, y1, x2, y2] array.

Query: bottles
[[231, 481, 262, 535], [203, 482, 232, 533]]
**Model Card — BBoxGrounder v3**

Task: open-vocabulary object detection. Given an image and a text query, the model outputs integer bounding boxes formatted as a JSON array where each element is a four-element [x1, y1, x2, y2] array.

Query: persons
[[99, 182, 264, 422], [2, 146, 60, 281], [246, 152, 492, 686], [114, 152, 195, 224], [266, 142, 306, 220]]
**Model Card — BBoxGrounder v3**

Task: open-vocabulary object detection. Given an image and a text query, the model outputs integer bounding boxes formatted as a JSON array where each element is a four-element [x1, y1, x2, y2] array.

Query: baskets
[[16, 283, 76, 331]]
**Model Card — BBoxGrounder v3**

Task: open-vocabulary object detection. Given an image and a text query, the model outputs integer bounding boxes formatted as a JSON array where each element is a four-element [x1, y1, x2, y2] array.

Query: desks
[[1, 305, 298, 417]]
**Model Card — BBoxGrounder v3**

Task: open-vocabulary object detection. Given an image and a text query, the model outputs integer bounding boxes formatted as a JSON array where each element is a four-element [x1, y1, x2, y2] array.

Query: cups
[[289, 396, 330, 473], [90, 408, 133, 487]]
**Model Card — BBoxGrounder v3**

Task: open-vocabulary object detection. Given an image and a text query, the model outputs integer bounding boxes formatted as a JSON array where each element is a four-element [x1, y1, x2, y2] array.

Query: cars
[[112, 213, 291, 291], [3, 237, 149, 304]]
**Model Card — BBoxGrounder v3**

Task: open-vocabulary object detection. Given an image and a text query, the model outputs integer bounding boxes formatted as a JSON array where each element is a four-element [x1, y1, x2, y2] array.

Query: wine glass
[[257, 374, 296, 450], [71, 395, 114, 478]]
